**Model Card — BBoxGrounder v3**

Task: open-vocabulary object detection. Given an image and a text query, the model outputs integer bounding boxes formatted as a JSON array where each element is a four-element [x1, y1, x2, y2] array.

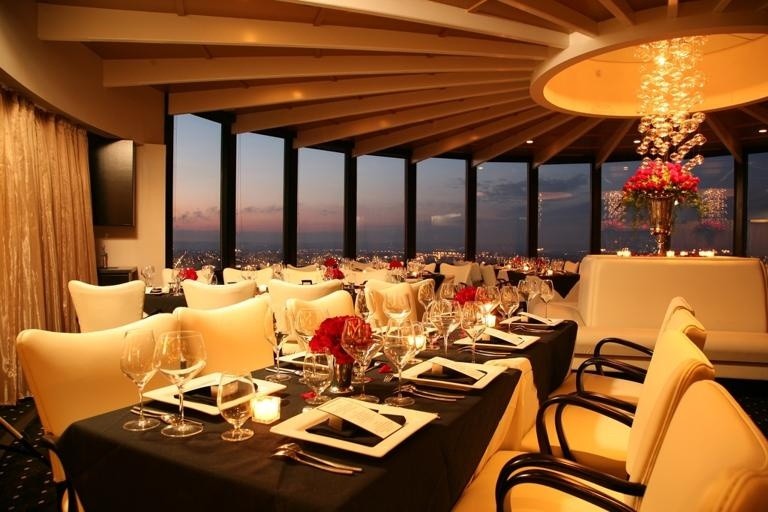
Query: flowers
[[622, 160, 701, 205]]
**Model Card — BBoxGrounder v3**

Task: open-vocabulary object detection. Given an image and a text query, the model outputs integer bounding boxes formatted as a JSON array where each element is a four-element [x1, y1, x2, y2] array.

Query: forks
[[268, 440, 363, 478]]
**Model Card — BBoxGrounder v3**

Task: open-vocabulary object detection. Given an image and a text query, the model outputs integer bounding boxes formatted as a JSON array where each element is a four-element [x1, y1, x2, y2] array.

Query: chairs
[[544, 296, 696, 408], [518, 309, 708, 483], [451, 330, 715, 512], [497, 378, 768, 512]]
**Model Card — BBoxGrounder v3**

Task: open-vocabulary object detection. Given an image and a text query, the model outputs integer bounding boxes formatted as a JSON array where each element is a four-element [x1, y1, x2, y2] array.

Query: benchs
[[528, 254, 768, 382]]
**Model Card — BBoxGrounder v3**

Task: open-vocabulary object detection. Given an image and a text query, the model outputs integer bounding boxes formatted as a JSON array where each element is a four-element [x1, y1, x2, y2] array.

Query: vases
[[646, 190, 679, 255]]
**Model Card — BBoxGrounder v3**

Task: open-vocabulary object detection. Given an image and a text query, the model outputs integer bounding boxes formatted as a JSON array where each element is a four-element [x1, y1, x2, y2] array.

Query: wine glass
[[139, 255, 215, 295], [218, 371, 255, 442], [243, 257, 565, 424], [153, 332, 208, 439], [120, 329, 161, 432]]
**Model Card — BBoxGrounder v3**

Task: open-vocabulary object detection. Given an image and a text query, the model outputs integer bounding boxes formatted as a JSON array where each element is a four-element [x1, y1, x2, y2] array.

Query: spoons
[[128, 409, 178, 425]]
